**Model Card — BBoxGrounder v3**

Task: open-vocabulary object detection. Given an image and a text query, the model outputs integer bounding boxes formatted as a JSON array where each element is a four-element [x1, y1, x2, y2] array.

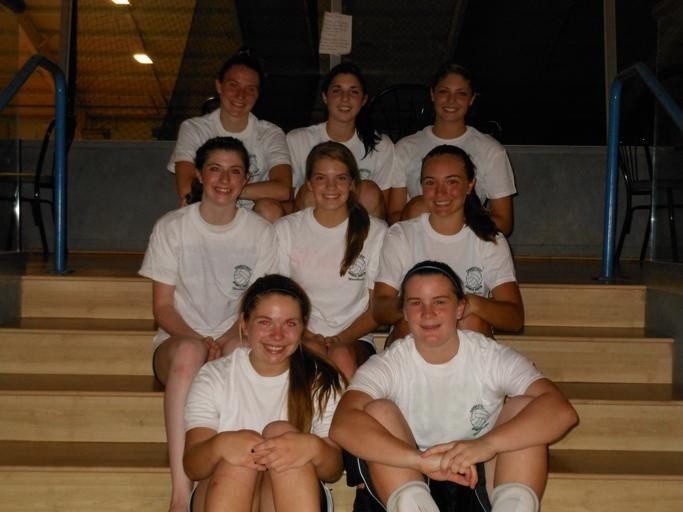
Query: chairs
[[0, 115, 78, 268], [355, 81, 432, 148], [614, 118, 683, 278]]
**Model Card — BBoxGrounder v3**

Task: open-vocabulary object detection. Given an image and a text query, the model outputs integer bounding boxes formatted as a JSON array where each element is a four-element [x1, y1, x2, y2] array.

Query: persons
[[136, 137, 278, 512], [371, 144, 525, 350], [271, 139, 388, 394], [328, 260, 579, 512], [387, 65, 519, 237], [183, 276, 347, 512], [286, 64, 395, 224], [165, 54, 291, 225]]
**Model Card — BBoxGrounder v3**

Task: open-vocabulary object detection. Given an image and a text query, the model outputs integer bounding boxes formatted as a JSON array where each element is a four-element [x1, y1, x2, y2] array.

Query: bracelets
[[329, 334, 342, 345]]
